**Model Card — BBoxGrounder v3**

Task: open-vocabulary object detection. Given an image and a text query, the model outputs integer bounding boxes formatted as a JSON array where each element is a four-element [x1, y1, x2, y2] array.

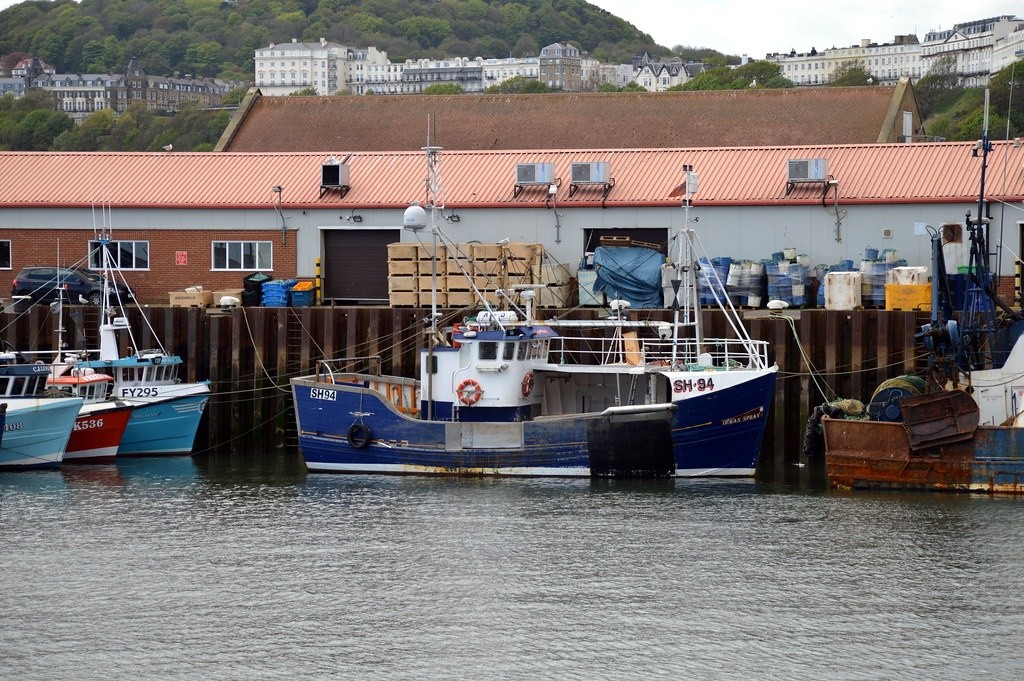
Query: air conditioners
[[320, 164, 349, 186], [787, 159, 828, 180], [570, 162, 609, 182], [516, 163, 554, 185]]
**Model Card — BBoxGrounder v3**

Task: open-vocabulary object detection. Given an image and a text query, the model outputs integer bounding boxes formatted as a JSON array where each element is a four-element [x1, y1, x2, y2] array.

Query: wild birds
[[77, 294, 88, 305], [162, 144, 173, 151], [749, 80, 756, 86], [866, 78, 873, 85]]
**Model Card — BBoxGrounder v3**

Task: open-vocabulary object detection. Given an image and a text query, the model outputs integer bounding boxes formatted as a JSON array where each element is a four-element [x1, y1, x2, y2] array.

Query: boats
[[822, 65, 1024, 496], [0, 177, 212, 466], [290, 113, 781, 484]]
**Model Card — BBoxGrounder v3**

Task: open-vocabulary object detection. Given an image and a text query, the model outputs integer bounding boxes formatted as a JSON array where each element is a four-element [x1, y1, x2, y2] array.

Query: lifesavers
[[522, 372, 534, 396], [458, 379, 481, 404], [347, 424, 372, 449]]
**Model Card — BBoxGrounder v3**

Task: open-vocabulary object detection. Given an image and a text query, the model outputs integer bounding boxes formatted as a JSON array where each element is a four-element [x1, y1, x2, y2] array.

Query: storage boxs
[[824, 272, 861, 310], [578, 236, 693, 308], [243, 272, 316, 306], [386, 242, 570, 308], [212, 289, 242, 305], [884, 266, 933, 311], [168, 291, 212, 308]]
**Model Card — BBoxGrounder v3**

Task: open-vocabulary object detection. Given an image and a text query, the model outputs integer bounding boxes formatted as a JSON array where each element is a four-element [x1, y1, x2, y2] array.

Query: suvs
[[14, 266, 137, 304]]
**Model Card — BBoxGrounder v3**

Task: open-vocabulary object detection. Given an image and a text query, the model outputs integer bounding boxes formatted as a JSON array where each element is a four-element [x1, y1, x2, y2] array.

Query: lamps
[[271, 186, 281, 192], [352, 214, 362, 222], [450, 215, 460, 222]]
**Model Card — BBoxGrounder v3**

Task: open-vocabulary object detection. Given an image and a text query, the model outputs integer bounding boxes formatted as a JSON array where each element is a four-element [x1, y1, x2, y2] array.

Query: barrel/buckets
[[956, 263, 976, 274], [697, 244, 908, 310], [867, 376, 936, 422]]
